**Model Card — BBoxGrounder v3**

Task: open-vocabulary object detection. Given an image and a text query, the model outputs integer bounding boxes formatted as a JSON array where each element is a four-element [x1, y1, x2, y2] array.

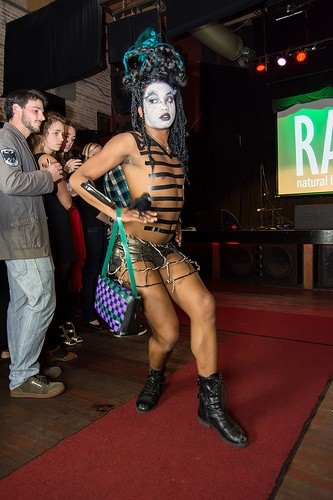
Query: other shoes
[[1, 348, 9, 358], [113, 327, 147, 338], [88, 320, 103, 331]]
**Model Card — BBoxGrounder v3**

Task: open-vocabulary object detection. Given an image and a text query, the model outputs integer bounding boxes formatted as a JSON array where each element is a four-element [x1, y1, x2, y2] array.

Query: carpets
[[0, 331, 333, 500]]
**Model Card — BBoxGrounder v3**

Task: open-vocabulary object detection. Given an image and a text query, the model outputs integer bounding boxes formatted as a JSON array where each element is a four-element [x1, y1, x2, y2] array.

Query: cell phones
[[78, 154, 86, 164]]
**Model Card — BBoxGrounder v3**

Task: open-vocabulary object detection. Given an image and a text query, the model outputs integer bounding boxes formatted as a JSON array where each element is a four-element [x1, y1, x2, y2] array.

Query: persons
[[73, 142, 107, 332], [69, 28, 249, 446], [29, 112, 86, 362], [0, 90, 65, 398]]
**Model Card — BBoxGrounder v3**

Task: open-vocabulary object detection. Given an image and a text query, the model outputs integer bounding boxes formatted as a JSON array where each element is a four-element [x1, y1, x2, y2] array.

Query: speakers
[[111, 71, 132, 115], [255, 242, 302, 287], [317, 244, 333, 288], [107, 8, 166, 64]]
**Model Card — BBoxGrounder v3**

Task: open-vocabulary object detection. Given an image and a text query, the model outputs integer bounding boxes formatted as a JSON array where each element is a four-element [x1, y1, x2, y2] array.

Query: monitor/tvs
[[274, 96, 333, 198]]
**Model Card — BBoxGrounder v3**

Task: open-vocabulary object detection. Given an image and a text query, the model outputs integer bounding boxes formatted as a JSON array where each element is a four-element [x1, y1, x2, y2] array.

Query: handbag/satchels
[[95, 274, 137, 334]]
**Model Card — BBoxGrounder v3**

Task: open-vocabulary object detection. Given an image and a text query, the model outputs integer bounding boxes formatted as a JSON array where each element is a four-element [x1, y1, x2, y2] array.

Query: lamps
[[295, 51, 312, 66], [277, 54, 289, 66]]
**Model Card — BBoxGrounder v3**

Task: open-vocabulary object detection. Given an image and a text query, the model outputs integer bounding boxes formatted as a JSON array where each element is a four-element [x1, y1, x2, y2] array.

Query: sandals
[[66, 322, 83, 343], [57, 325, 76, 346]]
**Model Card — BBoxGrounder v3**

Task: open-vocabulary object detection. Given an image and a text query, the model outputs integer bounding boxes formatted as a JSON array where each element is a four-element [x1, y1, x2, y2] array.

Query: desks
[[168, 228, 333, 291]]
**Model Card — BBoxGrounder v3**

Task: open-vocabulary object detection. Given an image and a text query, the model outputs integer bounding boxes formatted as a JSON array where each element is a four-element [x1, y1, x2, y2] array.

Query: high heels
[[46, 345, 79, 362]]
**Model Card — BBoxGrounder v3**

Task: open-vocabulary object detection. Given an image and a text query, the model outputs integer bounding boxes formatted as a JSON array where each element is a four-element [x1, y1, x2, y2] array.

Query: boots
[[198, 372, 247, 446], [136, 368, 165, 412]]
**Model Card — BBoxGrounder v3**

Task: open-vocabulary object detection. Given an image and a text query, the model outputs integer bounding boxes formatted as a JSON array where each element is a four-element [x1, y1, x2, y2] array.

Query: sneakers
[[9, 375, 65, 398], [40, 366, 62, 379]]
